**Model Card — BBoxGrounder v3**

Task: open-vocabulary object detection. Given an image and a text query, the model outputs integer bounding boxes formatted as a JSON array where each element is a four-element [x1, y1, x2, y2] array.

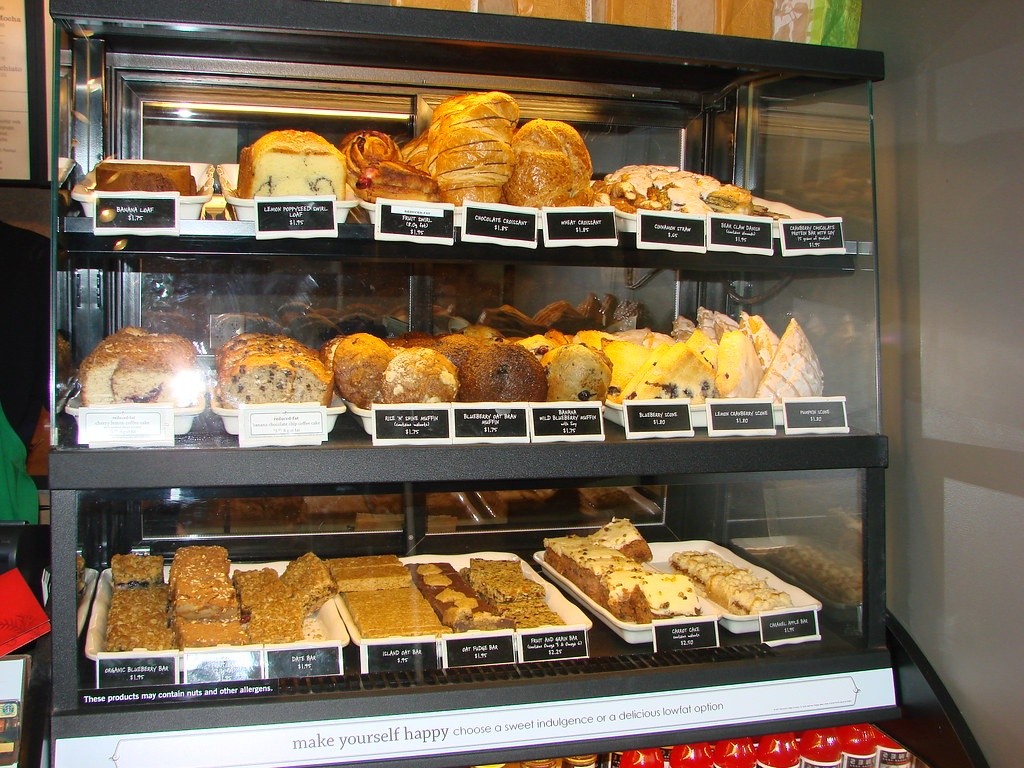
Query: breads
[[80, 91, 828, 655]]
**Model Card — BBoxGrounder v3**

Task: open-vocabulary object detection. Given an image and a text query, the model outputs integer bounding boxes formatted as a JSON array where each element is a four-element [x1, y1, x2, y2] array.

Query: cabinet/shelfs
[[27, 0, 988, 768]]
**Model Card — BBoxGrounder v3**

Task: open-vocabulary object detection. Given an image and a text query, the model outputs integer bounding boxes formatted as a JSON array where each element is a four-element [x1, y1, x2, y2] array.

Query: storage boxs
[[0, 655, 32, 768]]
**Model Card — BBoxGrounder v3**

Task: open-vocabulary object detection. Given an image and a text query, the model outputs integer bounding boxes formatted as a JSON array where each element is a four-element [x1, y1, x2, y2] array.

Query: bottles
[[466, 722, 917, 768]]
